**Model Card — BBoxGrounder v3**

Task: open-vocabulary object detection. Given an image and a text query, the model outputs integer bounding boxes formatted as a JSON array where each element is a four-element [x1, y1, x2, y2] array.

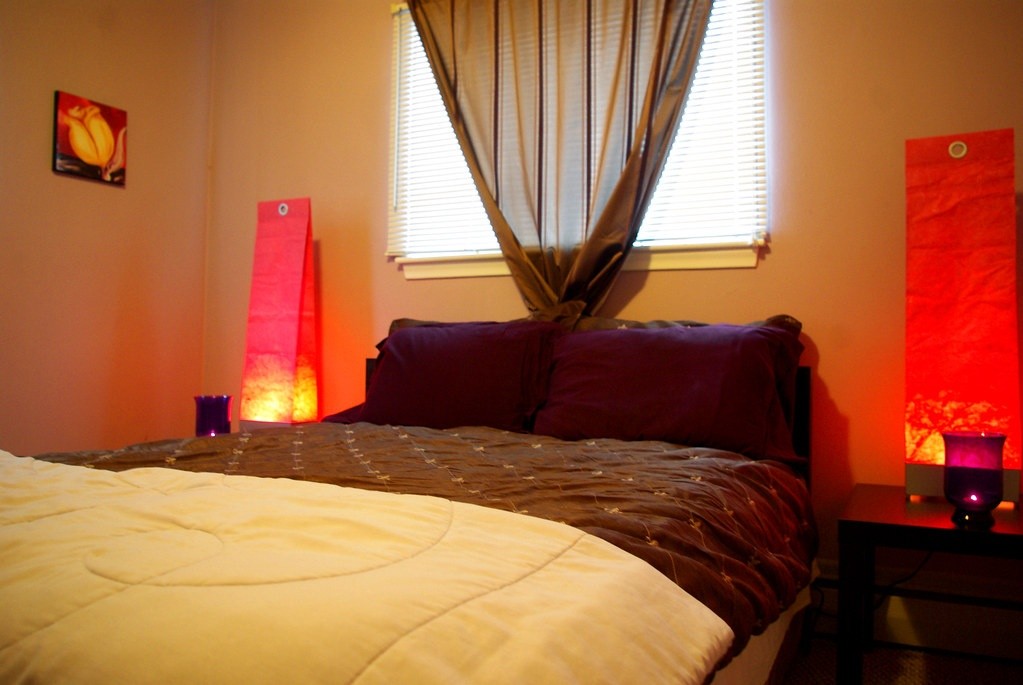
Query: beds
[[0, 418, 826, 685]]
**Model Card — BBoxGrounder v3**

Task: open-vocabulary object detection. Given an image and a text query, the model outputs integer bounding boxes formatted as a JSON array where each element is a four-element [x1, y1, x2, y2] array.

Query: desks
[[838, 484, 1023, 685]]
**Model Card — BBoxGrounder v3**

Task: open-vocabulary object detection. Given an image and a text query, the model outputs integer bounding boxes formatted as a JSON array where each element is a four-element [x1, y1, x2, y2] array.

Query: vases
[[940, 430, 1007, 534], [195, 396, 230, 438]]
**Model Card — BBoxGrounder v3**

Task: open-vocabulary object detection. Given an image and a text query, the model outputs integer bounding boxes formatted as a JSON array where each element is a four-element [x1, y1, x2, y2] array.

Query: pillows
[[325, 299, 809, 466]]
